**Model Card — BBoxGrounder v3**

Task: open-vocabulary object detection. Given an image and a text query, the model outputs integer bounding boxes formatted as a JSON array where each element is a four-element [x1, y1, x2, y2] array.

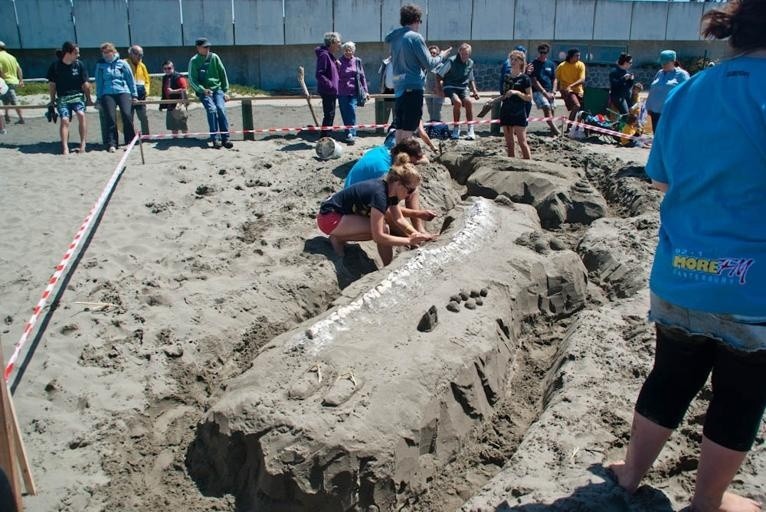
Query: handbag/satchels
[[170, 88, 190, 124], [416, 119, 452, 141], [354, 56, 369, 108]]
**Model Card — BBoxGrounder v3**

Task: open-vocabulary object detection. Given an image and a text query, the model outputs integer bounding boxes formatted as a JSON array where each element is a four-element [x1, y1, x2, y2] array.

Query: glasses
[[627, 61, 633, 65], [539, 50, 548, 55], [162, 67, 174, 70], [402, 183, 417, 195]]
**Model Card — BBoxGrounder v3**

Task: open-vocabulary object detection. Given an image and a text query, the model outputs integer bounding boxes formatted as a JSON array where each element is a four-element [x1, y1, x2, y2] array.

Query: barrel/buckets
[[315, 136, 344, 159]]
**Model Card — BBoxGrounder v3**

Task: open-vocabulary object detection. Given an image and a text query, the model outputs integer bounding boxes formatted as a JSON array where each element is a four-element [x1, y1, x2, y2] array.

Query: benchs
[[0, 92, 580, 144]]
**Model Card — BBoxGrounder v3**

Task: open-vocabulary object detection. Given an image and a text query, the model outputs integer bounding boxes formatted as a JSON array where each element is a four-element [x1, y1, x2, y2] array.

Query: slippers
[[44, 105, 59, 124]]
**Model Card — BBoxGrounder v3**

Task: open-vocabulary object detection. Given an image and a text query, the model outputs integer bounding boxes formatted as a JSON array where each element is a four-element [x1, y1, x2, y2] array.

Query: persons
[[317, 153, 438, 266], [158, 60, 188, 139], [45, 40, 94, 155], [120, 45, 151, 135], [343, 135, 437, 248], [611, 0, 766, 512], [0, 77, 10, 135], [0, 41, 25, 125], [187, 37, 234, 149], [645, 49, 690, 134], [95, 42, 138, 152]]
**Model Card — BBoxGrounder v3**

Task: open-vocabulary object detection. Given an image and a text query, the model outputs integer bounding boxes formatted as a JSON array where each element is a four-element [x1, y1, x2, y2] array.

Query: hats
[[655, 49, 678, 65], [634, 82, 644, 91], [0, 40, 6, 47], [194, 36, 212, 49]]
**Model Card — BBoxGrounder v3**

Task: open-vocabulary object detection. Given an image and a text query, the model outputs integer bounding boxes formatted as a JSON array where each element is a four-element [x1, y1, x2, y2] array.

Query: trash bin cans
[[582, 85, 611, 117]]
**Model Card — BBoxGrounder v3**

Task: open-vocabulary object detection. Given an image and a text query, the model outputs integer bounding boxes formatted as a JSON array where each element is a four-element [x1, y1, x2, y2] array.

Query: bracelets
[[403, 224, 411, 233]]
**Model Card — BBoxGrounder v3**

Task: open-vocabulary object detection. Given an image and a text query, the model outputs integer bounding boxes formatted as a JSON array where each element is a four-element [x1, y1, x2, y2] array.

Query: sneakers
[[107, 145, 118, 153], [123, 143, 131, 152], [221, 138, 234, 149], [450, 127, 461, 139], [0, 115, 25, 135], [211, 137, 223, 148], [465, 127, 476, 141]]
